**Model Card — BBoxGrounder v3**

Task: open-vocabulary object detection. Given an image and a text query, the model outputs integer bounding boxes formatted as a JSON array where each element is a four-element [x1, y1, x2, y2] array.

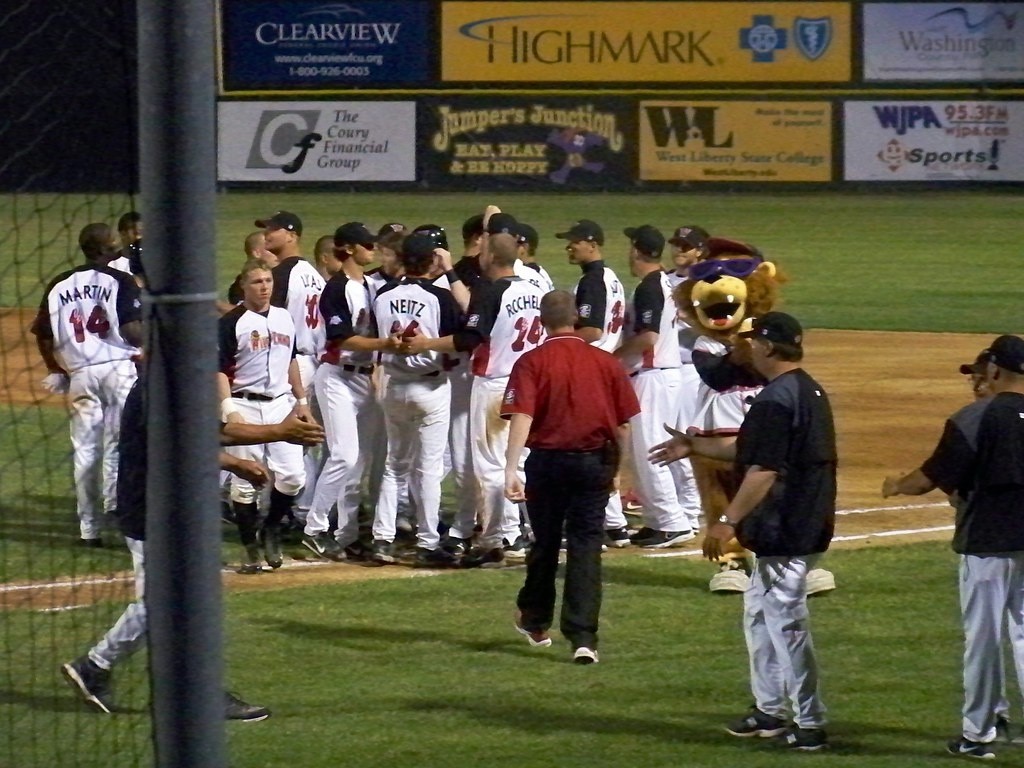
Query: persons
[[62, 380, 326, 723], [217, 205, 553, 572], [647, 311, 838, 750], [32, 212, 146, 546], [882, 334, 1024, 759], [500, 289, 641, 663], [557, 220, 712, 548]]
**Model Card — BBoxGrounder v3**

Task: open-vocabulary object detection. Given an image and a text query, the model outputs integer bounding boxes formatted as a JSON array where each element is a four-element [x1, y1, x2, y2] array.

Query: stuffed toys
[[672, 236, 836, 595]]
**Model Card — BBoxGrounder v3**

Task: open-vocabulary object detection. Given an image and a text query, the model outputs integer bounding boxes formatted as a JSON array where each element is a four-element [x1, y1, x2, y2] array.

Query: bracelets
[[299, 397, 308, 405], [446, 269, 460, 284]]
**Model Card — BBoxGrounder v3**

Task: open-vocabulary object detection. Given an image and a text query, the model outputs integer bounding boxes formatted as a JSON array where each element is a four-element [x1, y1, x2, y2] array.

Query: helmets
[[414, 224, 449, 249], [128, 240, 148, 275]]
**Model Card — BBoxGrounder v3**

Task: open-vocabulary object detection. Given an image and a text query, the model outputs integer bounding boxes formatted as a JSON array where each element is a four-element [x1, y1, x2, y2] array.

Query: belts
[[629, 368, 668, 377], [344, 363, 374, 374], [231, 392, 284, 401], [422, 371, 439, 377]]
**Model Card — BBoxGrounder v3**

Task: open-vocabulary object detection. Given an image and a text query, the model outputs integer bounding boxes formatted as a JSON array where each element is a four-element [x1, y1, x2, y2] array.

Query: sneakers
[[218, 489, 701, 570], [223, 690, 272, 723], [512, 611, 554, 648], [573, 647, 599, 663], [946, 735, 996, 761], [720, 703, 827, 751], [60, 655, 114, 714]]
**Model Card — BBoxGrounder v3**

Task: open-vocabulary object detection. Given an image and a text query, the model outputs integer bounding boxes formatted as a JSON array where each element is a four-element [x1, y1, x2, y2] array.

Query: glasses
[[361, 244, 376, 251]]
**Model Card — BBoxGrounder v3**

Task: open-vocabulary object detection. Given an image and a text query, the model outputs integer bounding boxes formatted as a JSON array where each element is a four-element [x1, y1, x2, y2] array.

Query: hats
[[378, 223, 407, 241], [959, 348, 992, 375], [981, 335, 1024, 375], [334, 222, 381, 246], [623, 225, 665, 257], [738, 311, 802, 348], [555, 220, 605, 246], [518, 223, 540, 248], [667, 224, 711, 249], [462, 214, 482, 238], [482, 213, 520, 237], [254, 211, 303, 238]]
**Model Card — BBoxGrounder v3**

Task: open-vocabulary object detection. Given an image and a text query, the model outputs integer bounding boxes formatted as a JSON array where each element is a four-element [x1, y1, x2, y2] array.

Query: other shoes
[[75, 539, 102, 548]]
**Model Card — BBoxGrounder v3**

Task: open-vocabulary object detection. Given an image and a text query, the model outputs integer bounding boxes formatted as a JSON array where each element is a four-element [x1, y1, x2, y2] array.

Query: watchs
[[718, 514, 738, 529]]
